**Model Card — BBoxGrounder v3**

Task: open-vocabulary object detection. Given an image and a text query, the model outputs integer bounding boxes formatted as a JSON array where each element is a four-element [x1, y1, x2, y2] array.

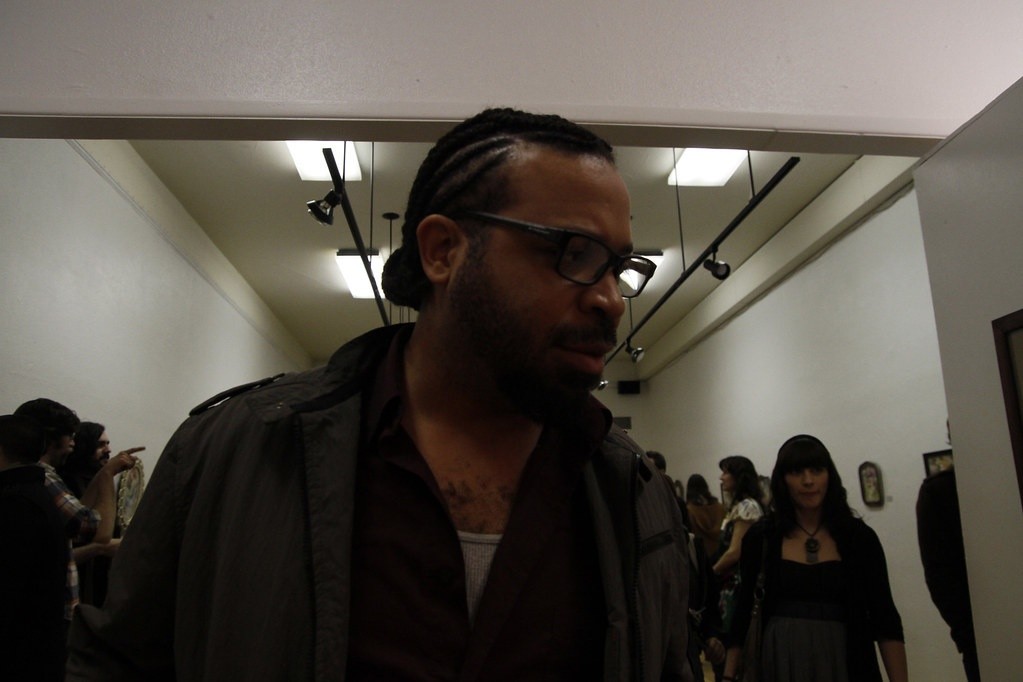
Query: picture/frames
[[114, 459, 146, 526], [859, 462, 885, 508], [922, 448, 954, 476], [989, 309, 1023, 511]]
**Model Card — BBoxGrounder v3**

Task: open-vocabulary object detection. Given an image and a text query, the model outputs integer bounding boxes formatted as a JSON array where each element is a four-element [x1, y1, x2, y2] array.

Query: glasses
[[451, 210, 657, 298]]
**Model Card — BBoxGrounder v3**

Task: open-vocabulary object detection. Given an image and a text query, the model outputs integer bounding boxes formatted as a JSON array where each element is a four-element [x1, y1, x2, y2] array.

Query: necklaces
[[797, 519, 825, 561]]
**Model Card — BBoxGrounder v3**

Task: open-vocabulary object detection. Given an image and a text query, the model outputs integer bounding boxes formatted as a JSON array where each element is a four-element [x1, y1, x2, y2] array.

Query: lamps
[[703, 248, 730, 280], [335, 248, 387, 299], [623, 337, 648, 361], [306, 188, 342, 226], [598, 378, 610, 391]]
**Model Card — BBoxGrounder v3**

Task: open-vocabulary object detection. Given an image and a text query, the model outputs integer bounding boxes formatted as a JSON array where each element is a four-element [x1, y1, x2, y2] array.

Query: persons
[[62, 108, 691, 682], [0, 397, 145, 681], [635, 432, 908, 680], [916, 413, 982, 682]]
